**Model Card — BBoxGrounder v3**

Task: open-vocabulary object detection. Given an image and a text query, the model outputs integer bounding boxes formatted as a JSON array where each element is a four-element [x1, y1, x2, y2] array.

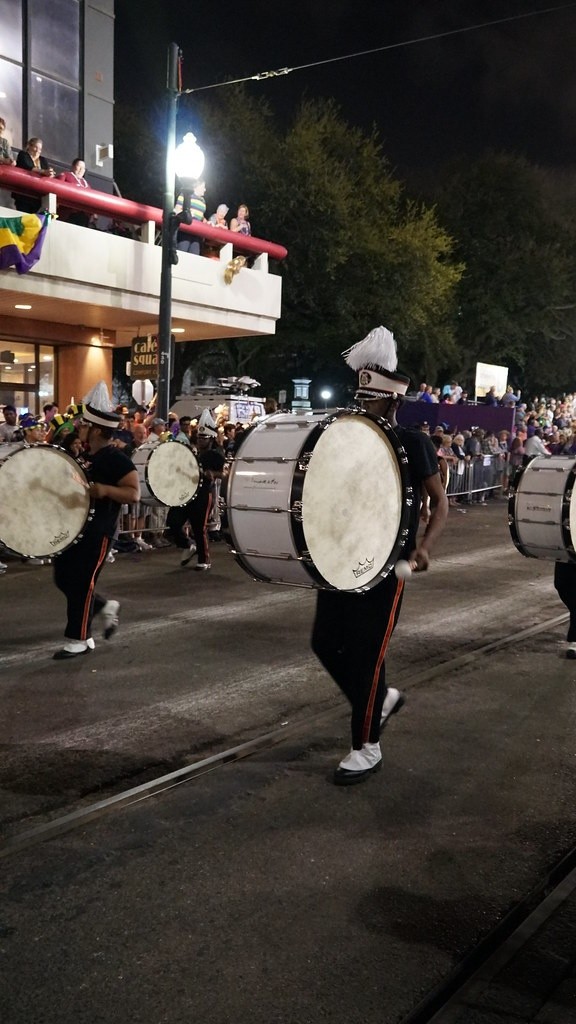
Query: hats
[[197, 409, 218, 438], [22, 419, 42, 430], [472, 428, 487, 435], [498, 429, 509, 435], [48, 413, 75, 440], [66, 404, 84, 420], [436, 426, 444, 432], [341, 325, 410, 400], [81, 380, 121, 429], [147, 418, 166, 429]]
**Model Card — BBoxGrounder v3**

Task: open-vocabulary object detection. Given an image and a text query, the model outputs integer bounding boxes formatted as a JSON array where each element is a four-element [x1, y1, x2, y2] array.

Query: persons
[[304, 326, 447, 786], [52, 380, 122, 659], [168, 408, 226, 570], [0, 117, 97, 228], [525, 398, 576, 658], [0, 380, 576, 574], [230, 205, 251, 268], [175, 179, 229, 261]]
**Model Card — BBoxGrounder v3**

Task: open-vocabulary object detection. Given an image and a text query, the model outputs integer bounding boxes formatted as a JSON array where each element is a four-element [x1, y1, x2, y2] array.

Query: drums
[[1, 440, 96, 560], [215, 406, 414, 595], [130, 439, 204, 508], [506, 454, 576, 565]]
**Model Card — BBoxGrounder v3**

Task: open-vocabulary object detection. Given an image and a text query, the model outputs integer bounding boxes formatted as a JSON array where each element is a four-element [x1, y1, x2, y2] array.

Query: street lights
[[158, 37, 207, 431], [320, 390, 332, 409]]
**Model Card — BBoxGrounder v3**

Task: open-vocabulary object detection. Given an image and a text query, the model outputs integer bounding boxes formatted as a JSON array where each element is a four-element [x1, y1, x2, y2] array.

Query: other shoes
[[195, 562, 212, 571], [333, 742, 384, 782], [566, 642, 576, 659], [0, 562, 8, 574], [100, 600, 120, 640], [52, 637, 95, 659], [379, 686, 406, 734], [180, 544, 197, 566], [105, 529, 222, 563], [24, 557, 45, 565]]
